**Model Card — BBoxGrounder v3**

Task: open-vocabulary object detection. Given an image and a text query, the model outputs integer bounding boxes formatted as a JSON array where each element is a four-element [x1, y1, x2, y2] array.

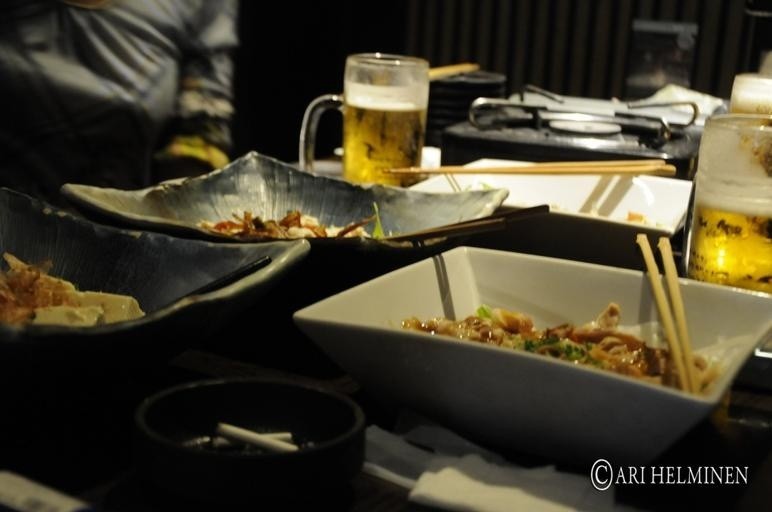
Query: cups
[[691, 112, 772, 290], [295, 54, 432, 188]]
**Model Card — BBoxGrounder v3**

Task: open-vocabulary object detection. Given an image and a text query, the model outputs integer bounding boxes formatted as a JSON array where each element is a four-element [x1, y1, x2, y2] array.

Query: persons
[[0, 0, 239, 204]]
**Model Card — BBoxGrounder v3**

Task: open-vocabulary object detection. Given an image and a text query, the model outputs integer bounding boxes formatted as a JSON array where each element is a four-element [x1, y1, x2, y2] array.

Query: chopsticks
[[634, 230, 702, 393], [371, 206, 549, 244], [386, 158, 678, 178]]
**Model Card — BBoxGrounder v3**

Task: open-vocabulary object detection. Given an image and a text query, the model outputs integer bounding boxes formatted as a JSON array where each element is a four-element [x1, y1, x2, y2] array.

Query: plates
[[2, 148, 772, 466]]
